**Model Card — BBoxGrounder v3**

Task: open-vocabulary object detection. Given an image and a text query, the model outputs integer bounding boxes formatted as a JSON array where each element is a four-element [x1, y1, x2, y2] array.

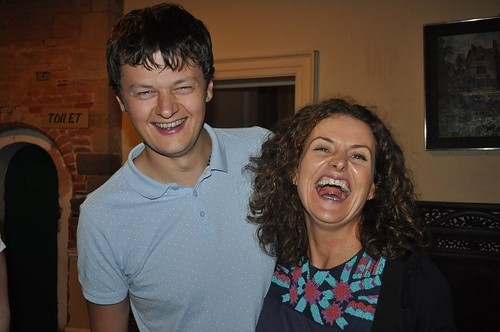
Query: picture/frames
[[423, 16, 500, 152]]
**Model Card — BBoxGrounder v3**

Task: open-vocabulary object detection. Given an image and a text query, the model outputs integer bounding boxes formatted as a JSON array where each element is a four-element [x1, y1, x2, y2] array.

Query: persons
[[247, 98, 455, 332], [76, 4, 278, 332]]
